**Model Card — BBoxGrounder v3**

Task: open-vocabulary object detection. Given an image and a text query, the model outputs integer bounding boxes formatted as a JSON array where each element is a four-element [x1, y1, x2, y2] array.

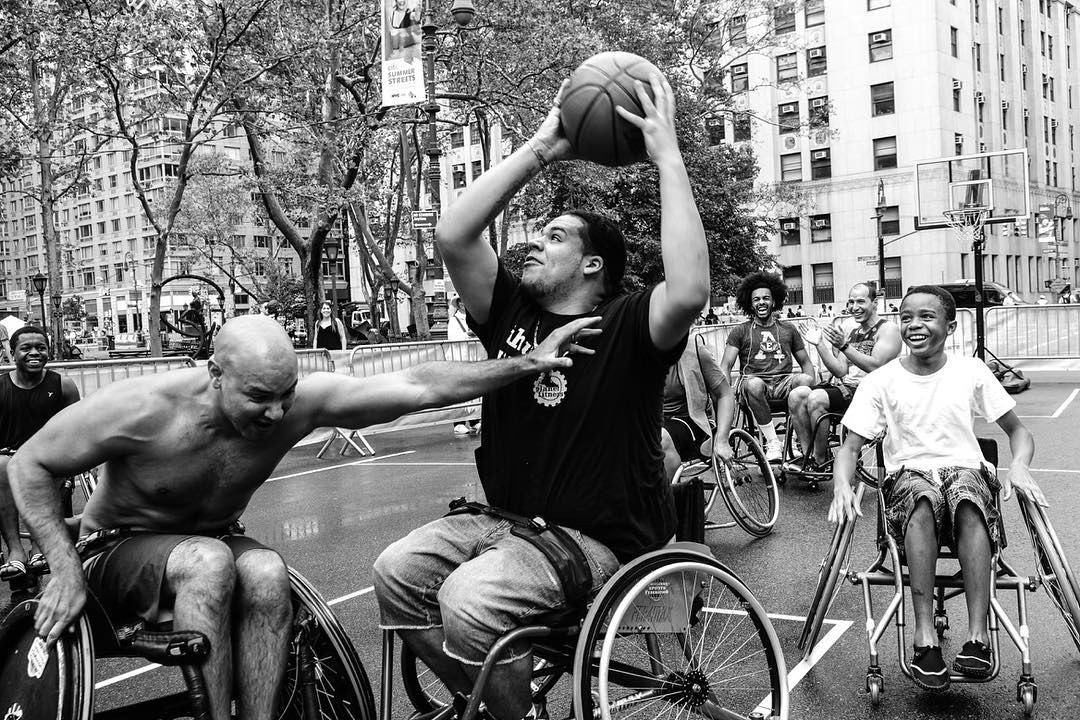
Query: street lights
[[382, 282, 394, 342], [389, 274, 403, 342], [216, 294, 226, 326], [323, 234, 340, 318], [51, 290, 63, 359], [1052, 193, 1074, 305], [869, 178, 887, 295], [32, 270, 49, 334], [419, 0, 477, 340], [122, 251, 144, 353], [209, 240, 236, 317]]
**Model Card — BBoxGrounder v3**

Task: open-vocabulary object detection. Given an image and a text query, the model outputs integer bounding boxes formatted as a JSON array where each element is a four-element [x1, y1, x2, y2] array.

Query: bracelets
[[527, 142, 547, 170], [837, 343, 849, 351]]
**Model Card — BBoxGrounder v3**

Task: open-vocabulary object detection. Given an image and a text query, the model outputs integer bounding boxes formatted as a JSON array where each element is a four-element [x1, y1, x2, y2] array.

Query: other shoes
[[762, 440, 785, 463], [779, 456, 833, 479], [785, 436, 804, 460], [453, 421, 481, 436], [450, 690, 549, 720]]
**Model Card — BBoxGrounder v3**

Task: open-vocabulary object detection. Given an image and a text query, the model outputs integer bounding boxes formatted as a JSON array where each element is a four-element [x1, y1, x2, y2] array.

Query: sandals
[[29, 553, 50, 572], [0, 561, 28, 581]]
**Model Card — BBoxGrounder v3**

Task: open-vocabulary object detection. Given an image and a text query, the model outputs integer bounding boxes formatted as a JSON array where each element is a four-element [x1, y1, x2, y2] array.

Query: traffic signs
[[411, 210, 439, 229]]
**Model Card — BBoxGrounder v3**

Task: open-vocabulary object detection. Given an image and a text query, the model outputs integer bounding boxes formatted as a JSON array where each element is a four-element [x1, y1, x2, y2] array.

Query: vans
[[906, 279, 1027, 309]]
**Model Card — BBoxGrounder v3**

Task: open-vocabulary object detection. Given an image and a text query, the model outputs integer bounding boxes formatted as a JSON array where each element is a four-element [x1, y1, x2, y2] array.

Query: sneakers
[[912, 645, 951, 691], [954, 640, 994, 675]]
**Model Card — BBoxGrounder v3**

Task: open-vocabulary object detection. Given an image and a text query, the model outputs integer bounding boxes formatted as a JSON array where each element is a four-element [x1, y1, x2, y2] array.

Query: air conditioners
[[733, 64, 744, 78], [1051, 120, 1061, 128], [779, 99, 828, 115], [814, 151, 827, 159], [872, 32, 887, 42], [783, 219, 826, 231], [1042, 76, 1050, 85], [808, 47, 824, 58], [954, 136, 987, 153], [1022, 63, 1028, 73], [952, 80, 1009, 110], [1024, 108, 1031, 118]]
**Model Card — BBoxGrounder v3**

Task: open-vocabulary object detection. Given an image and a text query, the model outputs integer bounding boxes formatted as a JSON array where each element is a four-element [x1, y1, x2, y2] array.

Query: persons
[[660, 333, 735, 481], [783, 283, 903, 475], [692, 299, 738, 326], [772, 300, 851, 320], [371, 73, 709, 720], [720, 271, 814, 466], [889, 303, 898, 312], [446, 292, 481, 434], [1003, 289, 1080, 306], [827, 285, 1048, 689], [0, 312, 608, 720], [312, 301, 346, 350]]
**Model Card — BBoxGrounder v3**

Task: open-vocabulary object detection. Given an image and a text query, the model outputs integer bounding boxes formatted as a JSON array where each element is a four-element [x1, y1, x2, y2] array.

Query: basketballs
[[560, 51, 667, 168]]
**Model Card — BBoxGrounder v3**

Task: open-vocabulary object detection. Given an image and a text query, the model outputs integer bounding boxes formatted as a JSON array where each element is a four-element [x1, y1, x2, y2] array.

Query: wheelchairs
[[0, 445, 100, 592], [795, 437, 1080, 714], [381, 475, 790, 720], [670, 364, 881, 539], [0, 517, 378, 720]]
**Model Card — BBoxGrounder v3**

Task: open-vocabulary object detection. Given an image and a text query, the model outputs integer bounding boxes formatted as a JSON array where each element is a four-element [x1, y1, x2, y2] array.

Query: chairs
[[59, 342, 83, 361]]
